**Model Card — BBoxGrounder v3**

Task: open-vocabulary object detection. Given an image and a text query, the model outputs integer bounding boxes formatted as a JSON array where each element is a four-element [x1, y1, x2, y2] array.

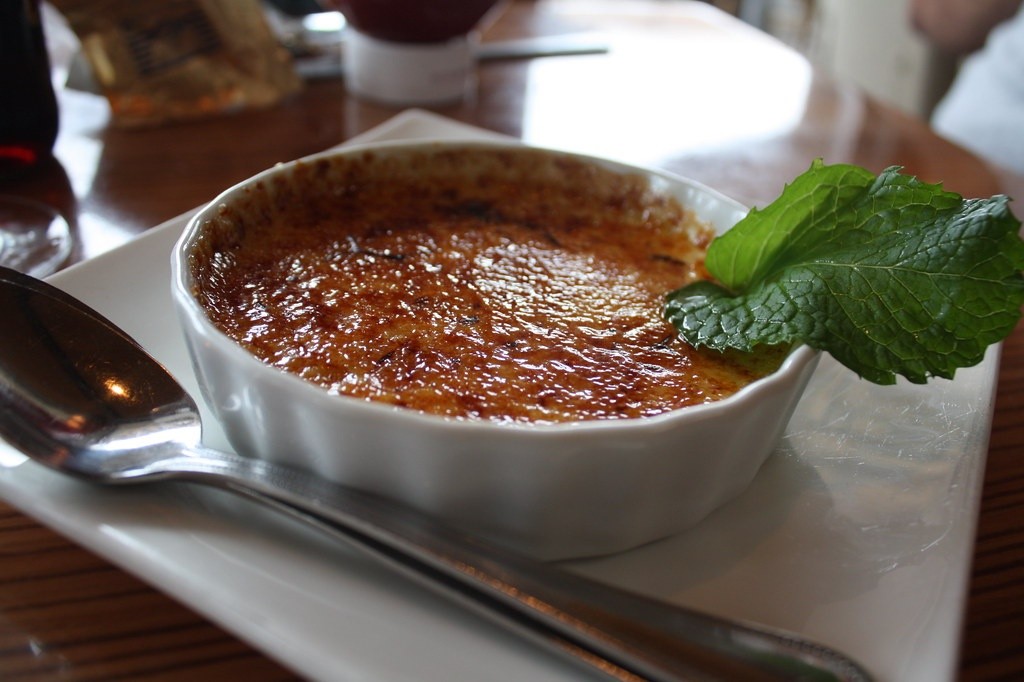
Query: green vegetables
[[661, 154, 1024, 387]]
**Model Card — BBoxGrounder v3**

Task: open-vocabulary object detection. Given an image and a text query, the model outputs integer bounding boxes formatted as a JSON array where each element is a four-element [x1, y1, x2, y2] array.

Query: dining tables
[[1, 1, 1024, 682]]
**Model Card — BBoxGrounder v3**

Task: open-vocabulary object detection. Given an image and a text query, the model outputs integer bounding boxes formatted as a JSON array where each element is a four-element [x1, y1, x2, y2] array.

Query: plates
[[0, 107, 1001, 682]]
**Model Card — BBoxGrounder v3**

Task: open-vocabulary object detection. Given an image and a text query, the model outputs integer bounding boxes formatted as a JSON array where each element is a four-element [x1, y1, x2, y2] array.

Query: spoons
[[1, 268, 876, 681]]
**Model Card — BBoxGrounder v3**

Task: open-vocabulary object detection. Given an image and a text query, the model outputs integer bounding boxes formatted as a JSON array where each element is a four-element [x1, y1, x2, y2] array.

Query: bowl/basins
[[171, 137, 820, 563]]
